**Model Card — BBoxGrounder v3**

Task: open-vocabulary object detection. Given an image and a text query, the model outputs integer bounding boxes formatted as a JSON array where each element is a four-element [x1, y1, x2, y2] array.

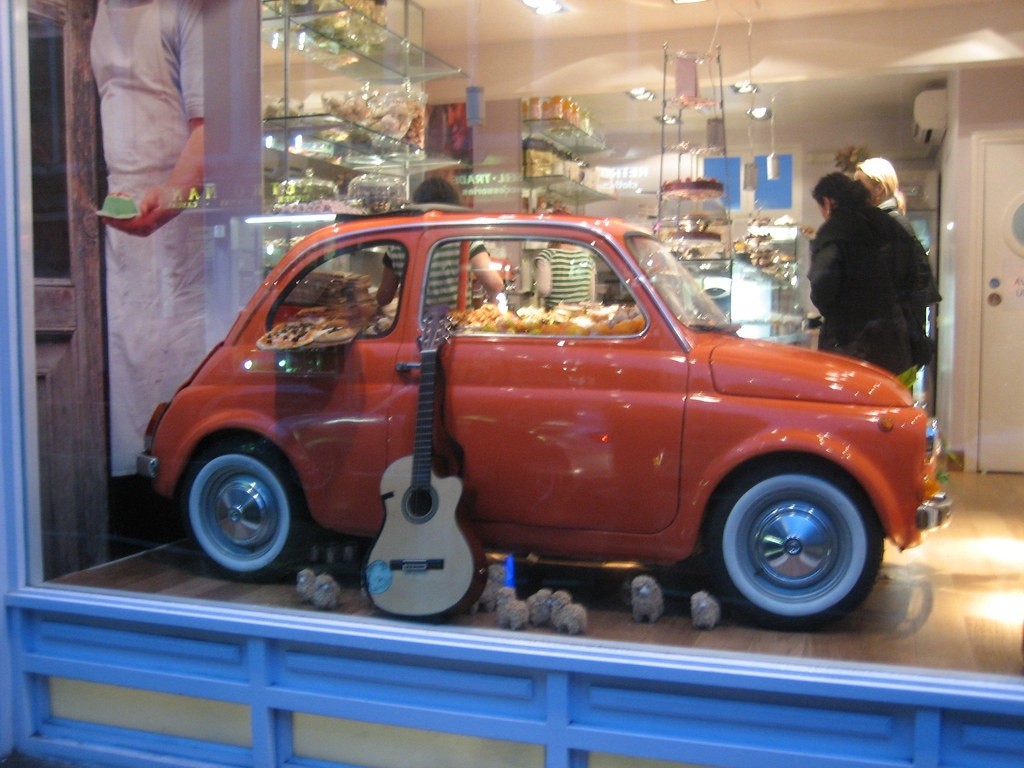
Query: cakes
[[346, 178, 406, 203], [278, 183, 334, 204]]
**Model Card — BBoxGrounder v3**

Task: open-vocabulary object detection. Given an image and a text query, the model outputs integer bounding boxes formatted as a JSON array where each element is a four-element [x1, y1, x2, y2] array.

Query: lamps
[[767, 96, 780, 180], [743, 115, 757, 191], [465, 0, 486, 127]]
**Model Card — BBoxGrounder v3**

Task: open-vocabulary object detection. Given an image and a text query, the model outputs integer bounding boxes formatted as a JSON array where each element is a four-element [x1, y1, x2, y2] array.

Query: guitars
[[363, 304, 492, 621]]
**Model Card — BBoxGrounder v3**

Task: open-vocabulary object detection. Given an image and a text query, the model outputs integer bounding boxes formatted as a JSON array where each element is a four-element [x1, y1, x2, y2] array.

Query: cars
[[138, 212, 948, 632]]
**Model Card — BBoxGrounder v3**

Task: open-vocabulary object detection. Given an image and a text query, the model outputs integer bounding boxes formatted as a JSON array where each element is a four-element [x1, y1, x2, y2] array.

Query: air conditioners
[[909, 88, 948, 148]]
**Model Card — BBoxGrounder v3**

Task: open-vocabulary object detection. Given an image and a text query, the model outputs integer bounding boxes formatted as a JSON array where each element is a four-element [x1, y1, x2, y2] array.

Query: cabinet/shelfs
[[257, 0, 798, 345]]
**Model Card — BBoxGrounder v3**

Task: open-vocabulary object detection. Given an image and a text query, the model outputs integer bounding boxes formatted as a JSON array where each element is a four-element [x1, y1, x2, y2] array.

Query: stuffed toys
[[527, 588, 553, 626], [691, 591, 721, 631], [550, 591, 587, 634], [314, 575, 340, 609], [470, 564, 505, 614], [497, 588, 528, 630], [296, 570, 316, 603], [631, 576, 665, 623]]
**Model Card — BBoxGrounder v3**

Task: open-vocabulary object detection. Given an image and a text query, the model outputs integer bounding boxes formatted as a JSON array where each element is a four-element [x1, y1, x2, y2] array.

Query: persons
[[88, 0, 204, 479], [807, 171, 931, 395], [853, 157, 942, 305], [375, 178, 503, 313], [534, 211, 596, 310]]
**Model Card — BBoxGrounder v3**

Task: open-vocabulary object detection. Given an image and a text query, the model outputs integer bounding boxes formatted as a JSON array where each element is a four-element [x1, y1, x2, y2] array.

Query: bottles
[[523, 94, 593, 187]]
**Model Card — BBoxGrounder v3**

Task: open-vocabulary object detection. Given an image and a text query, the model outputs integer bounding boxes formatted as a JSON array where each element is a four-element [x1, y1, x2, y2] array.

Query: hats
[[333, 174, 421, 217]]
